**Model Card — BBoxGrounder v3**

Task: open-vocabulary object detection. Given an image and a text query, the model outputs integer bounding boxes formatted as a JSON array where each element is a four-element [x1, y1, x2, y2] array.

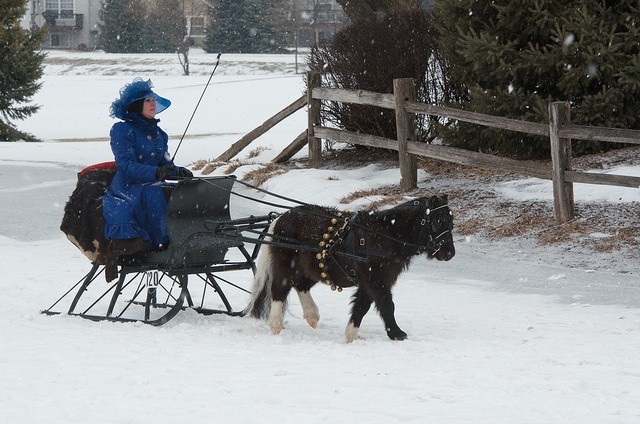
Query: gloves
[[157, 165, 174, 178], [178, 167, 193, 178]]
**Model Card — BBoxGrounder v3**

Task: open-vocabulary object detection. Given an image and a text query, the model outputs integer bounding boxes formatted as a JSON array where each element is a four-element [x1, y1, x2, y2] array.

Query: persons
[[101, 77, 193, 251]]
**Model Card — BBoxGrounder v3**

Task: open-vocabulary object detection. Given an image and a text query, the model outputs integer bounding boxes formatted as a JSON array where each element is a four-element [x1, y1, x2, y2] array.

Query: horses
[[242, 191, 458, 344]]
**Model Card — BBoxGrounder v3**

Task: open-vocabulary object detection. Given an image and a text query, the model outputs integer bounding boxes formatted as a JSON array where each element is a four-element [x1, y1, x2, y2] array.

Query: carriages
[[39, 162, 455, 340]]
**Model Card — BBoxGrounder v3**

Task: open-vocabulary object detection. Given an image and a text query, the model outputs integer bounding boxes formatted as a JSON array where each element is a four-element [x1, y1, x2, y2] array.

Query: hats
[[109, 77, 171, 118]]
